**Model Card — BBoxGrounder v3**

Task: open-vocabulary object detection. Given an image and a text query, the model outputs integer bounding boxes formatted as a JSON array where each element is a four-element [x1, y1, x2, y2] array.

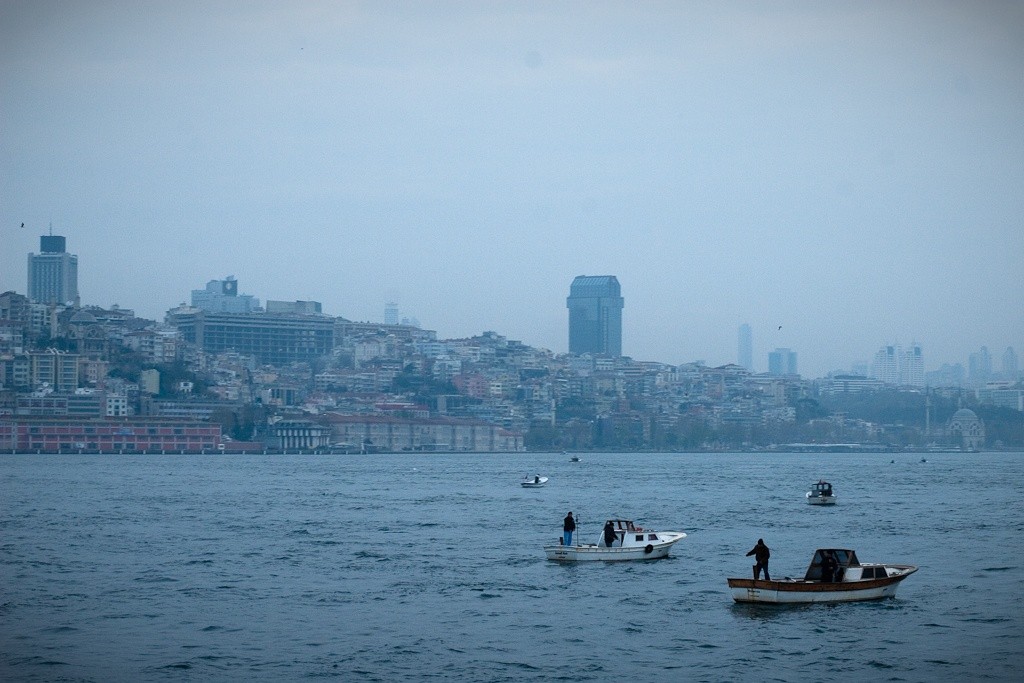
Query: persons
[[745, 538, 770, 580], [535, 473, 540, 483], [603, 522, 619, 547], [563, 512, 576, 546]]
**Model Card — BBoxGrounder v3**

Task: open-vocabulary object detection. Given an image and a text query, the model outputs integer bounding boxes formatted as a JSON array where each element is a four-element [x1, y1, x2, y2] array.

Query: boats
[[543, 520, 687, 560], [727, 549, 918, 603], [568, 454, 585, 464], [806, 480, 838, 504], [521, 473, 550, 489]]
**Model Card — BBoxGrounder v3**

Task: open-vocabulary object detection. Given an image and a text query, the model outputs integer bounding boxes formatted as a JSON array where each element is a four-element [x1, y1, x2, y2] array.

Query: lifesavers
[[645, 544, 653, 553]]
